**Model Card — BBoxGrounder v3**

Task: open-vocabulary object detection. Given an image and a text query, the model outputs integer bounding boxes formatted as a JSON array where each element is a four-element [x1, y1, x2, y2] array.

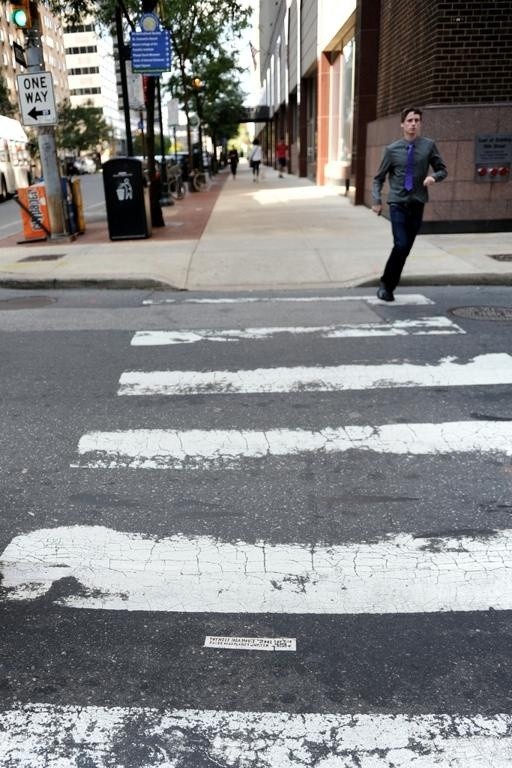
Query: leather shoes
[[377, 286, 395, 302]]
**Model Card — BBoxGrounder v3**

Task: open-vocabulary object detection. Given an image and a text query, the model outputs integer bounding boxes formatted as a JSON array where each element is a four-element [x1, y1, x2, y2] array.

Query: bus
[[0, 114, 36, 203]]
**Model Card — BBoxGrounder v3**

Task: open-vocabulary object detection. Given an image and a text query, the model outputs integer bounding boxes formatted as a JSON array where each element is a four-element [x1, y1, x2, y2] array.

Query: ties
[[404, 143, 414, 193]]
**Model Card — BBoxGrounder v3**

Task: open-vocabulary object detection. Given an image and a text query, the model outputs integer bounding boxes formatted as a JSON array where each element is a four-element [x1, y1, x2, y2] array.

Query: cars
[[71, 157, 97, 174], [132, 150, 214, 185]]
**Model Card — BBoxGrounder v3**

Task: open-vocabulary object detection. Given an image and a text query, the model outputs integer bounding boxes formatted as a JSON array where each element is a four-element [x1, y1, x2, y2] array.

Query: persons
[[275, 135, 290, 179], [247, 137, 264, 183], [228, 144, 240, 182], [372, 105, 449, 302], [339, 143, 353, 197]]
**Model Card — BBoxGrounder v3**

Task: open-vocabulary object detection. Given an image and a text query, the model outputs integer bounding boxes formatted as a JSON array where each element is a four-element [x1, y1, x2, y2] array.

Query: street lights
[[191, 75, 208, 184]]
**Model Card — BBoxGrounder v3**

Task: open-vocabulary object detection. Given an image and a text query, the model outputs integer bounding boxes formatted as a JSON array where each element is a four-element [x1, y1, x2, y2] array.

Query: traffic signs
[[16, 71, 58, 127]]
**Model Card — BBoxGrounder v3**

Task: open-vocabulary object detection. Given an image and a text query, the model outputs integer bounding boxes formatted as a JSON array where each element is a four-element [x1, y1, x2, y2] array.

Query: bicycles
[[167, 162, 186, 201], [190, 150, 212, 192]]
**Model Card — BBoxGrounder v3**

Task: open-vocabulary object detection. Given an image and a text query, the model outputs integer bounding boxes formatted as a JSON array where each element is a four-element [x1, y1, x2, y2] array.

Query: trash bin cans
[[102, 158, 150, 241]]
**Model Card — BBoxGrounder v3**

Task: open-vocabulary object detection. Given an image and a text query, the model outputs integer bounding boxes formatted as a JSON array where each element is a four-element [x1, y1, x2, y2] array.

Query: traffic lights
[[10, 0, 33, 30]]
